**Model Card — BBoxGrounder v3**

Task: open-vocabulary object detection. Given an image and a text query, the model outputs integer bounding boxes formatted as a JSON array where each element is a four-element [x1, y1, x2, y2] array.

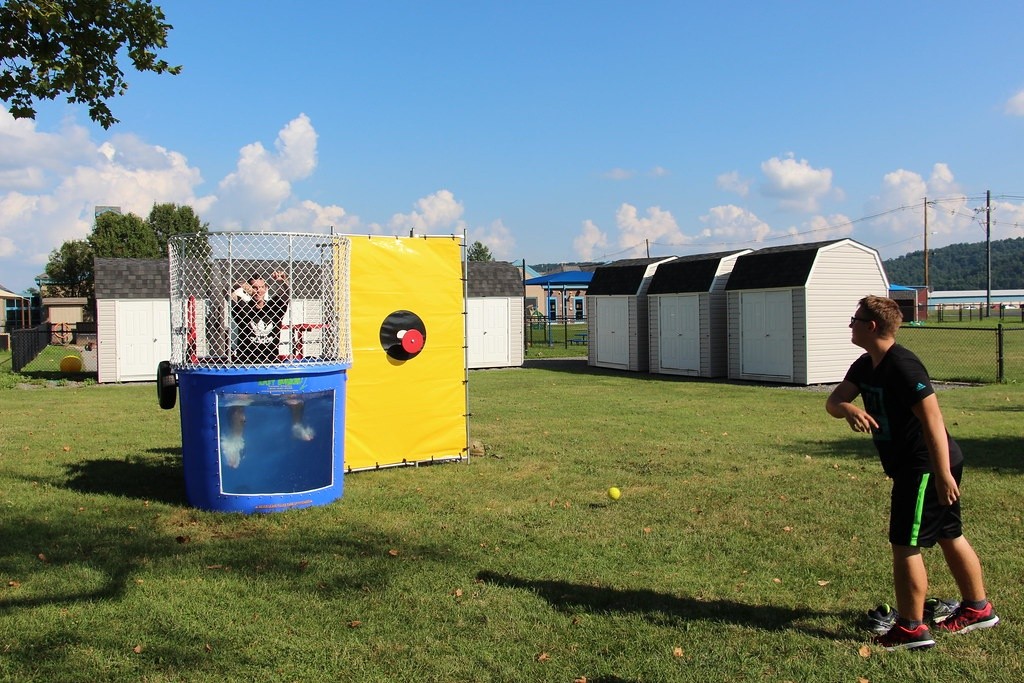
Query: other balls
[[607, 487, 621, 500]]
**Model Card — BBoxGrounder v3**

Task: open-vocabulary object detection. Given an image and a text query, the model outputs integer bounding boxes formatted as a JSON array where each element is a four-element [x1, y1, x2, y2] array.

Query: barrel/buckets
[[173, 362, 352, 515]]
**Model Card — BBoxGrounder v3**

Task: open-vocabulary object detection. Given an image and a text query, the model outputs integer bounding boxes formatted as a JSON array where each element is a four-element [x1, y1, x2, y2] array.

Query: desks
[[574, 334, 587, 345]]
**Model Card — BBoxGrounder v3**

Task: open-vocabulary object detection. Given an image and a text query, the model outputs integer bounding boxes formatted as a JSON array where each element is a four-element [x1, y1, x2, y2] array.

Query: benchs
[[568, 339, 589, 345]]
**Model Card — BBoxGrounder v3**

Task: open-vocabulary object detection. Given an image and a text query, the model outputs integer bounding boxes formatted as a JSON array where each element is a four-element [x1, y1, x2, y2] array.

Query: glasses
[[850, 316, 871, 326]]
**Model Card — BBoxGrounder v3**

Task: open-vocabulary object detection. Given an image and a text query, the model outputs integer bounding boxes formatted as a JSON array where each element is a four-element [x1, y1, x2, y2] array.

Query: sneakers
[[874, 622, 936, 649], [931, 603, 1002, 632], [924, 596, 962, 624], [862, 603, 900, 635]]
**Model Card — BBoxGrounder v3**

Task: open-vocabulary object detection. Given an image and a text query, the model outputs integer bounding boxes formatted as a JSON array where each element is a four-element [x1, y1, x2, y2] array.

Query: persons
[[826, 294, 1000, 652], [223, 271, 314, 364]]
